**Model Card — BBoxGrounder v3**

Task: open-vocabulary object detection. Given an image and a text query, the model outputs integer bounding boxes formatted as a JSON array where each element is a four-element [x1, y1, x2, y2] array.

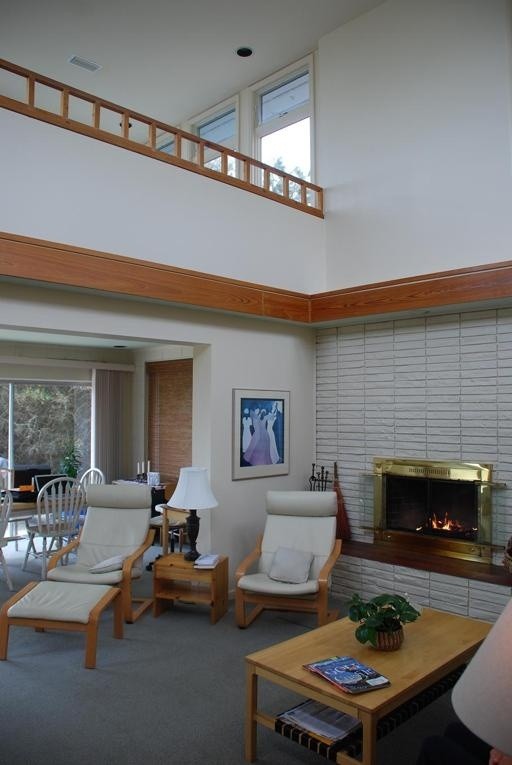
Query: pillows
[[87, 554, 129, 575], [269, 547, 314, 584]]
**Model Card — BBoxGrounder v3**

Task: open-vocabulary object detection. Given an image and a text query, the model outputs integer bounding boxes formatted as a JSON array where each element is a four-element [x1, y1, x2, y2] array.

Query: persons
[[241, 399, 280, 466], [417, 723, 509, 765]]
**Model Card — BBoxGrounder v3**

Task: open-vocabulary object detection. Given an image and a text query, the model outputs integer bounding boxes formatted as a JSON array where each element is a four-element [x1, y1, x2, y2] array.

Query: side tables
[[153, 551, 229, 624]]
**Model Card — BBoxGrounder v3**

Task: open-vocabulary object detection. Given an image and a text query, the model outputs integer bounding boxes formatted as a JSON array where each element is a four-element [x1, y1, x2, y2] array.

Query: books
[[193, 558, 220, 570], [280, 700, 363, 745], [309, 655, 390, 696], [194, 554, 219, 566], [303, 656, 350, 695]]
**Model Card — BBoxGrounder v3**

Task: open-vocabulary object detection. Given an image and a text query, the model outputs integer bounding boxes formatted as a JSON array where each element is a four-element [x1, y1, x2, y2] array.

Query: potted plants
[[347, 592, 422, 651]]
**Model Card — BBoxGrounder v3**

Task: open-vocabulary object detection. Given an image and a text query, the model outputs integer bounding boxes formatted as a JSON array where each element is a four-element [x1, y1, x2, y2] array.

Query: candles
[[137, 460, 151, 474]]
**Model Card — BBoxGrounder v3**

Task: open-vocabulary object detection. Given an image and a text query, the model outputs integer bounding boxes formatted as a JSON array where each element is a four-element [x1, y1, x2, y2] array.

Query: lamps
[[166, 466, 219, 561], [451, 596, 512, 757]]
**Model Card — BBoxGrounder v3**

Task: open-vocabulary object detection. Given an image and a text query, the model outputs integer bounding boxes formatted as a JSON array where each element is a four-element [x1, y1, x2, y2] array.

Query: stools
[[0, 580, 123, 670]]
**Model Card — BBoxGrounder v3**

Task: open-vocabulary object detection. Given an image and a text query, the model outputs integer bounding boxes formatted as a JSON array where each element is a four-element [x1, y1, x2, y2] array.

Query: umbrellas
[[330, 461, 351, 539]]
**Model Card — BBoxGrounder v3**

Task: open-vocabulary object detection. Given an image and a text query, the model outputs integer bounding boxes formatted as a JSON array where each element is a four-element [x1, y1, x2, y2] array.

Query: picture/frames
[[232, 388, 291, 481]]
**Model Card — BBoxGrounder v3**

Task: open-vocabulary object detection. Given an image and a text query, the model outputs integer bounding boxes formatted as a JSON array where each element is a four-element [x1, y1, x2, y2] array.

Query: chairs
[[0, 467, 105, 591], [235, 490, 342, 628], [46, 484, 156, 624], [150, 504, 190, 555]]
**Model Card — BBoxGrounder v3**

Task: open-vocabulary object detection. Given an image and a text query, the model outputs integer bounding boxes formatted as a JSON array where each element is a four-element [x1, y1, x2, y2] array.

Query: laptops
[[34, 474, 78, 501]]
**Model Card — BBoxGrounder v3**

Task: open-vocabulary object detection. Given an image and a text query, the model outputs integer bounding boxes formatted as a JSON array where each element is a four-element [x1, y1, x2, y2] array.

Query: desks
[[112, 480, 165, 518]]
